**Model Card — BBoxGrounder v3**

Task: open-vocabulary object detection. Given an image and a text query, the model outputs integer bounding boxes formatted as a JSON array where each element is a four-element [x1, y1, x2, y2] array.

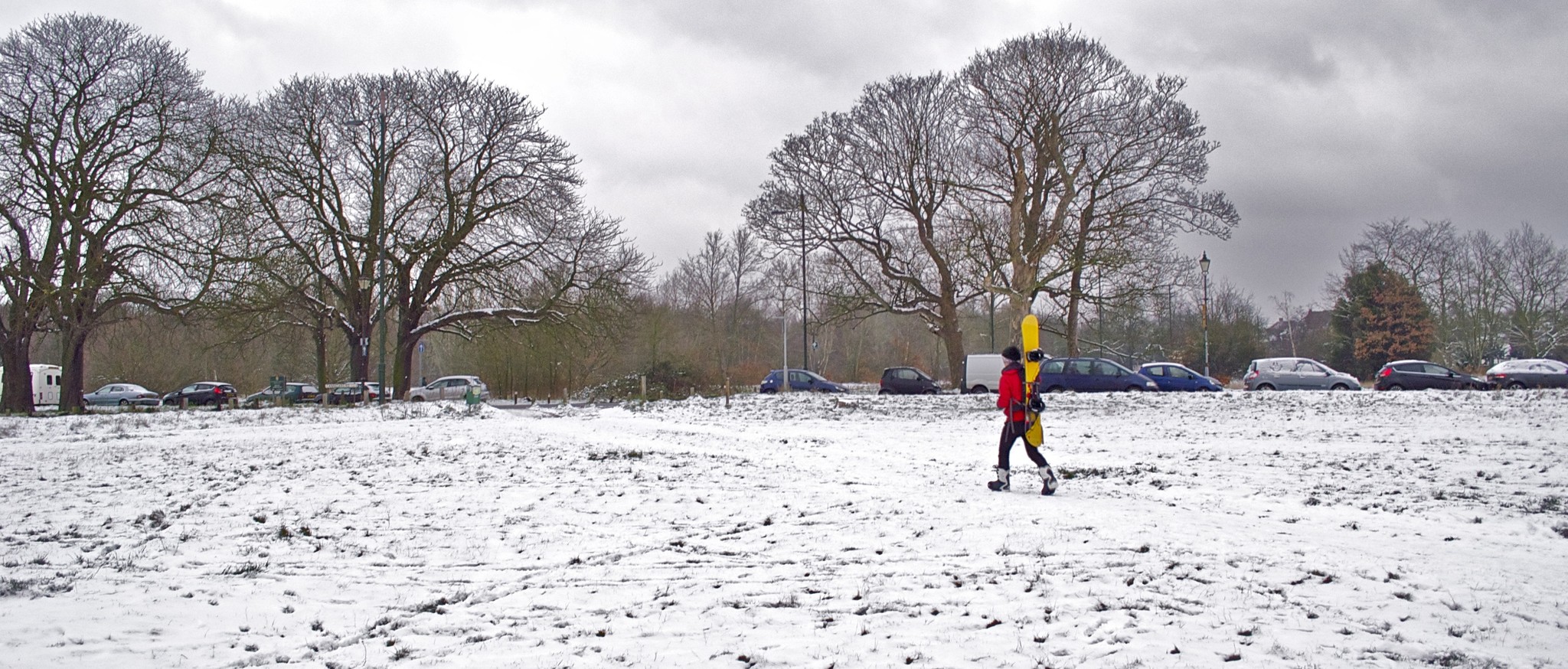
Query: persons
[[421, 377, 427, 387], [988, 347, 1058, 495]]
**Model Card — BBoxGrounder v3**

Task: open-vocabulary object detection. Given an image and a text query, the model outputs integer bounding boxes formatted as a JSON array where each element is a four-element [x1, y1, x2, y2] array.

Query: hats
[[1002, 346, 1021, 361]]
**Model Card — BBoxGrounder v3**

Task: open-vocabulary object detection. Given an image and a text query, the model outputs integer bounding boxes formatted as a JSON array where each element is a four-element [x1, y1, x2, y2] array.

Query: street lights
[[358, 272, 372, 402], [772, 316, 789, 391], [1199, 250, 1211, 377], [343, 89, 386, 406], [770, 188, 809, 370]]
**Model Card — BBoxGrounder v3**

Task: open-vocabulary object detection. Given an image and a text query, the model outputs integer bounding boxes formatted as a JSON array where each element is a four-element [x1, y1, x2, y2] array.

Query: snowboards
[[1021, 314, 1042, 447]]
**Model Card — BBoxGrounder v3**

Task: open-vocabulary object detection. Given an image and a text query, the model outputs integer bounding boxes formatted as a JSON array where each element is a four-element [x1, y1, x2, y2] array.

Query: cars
[[1242, 357, 1361, 391], [83, 383, 160, 406], [243, 383, 319, 410], [314, 382, 392, 405], [1373, 360, 1489, 391], [1486, 359, 1568, 390], [162, 381, 239, 406], [1138, 363, 1223, 392], [1038, 356, 1159, 393], [759, 369, 850, 395], [878, 366, 942, 395]]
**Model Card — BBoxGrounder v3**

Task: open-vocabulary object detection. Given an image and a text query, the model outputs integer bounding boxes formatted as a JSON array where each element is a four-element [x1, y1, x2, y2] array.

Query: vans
[[960, 352, 1064, 394]]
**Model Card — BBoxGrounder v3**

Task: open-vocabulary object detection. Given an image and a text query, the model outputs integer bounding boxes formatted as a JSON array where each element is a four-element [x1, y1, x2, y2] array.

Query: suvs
[[403, 375, 489, 403]]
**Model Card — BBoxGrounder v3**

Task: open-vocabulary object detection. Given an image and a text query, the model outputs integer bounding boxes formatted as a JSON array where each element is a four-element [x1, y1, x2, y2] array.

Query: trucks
[[0, 363, 62, 405]]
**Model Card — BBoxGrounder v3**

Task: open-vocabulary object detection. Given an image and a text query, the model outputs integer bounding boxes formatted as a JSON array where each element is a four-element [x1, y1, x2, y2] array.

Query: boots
[[1037, 465, 1058, 495], [987, 465, 1011, 492]]
